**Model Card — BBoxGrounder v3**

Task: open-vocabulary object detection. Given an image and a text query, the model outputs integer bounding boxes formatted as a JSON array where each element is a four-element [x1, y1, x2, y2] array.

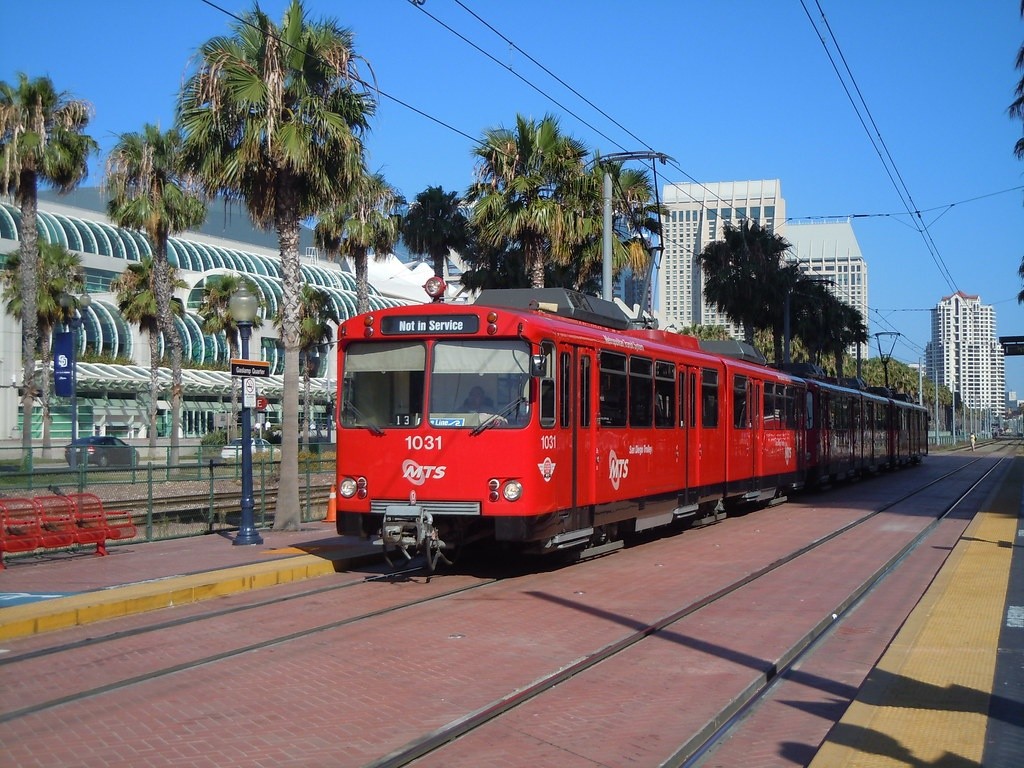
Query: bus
[[305, 275, 932, 575]]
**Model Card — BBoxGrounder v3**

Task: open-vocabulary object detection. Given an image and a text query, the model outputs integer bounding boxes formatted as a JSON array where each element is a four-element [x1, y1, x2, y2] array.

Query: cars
[[220, 438, 280, 460], [65, 436, 140, 469]]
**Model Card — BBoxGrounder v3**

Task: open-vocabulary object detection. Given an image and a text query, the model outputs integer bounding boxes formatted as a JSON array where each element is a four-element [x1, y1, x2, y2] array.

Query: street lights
[[58, 292, 91, 468], [225, 276, 264, 546]]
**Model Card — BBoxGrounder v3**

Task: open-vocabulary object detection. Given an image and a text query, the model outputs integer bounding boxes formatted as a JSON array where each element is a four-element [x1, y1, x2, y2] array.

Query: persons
[[463, 386, 493, 413], [969, 432, 977, 452]]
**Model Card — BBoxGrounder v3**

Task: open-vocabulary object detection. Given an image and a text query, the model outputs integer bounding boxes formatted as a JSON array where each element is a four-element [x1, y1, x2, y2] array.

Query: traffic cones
[[320, 483, 337, 522]]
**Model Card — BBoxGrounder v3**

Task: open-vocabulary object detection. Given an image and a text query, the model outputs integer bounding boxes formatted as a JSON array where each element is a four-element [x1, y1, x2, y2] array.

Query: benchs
[[0, 493, 137, 570]]
